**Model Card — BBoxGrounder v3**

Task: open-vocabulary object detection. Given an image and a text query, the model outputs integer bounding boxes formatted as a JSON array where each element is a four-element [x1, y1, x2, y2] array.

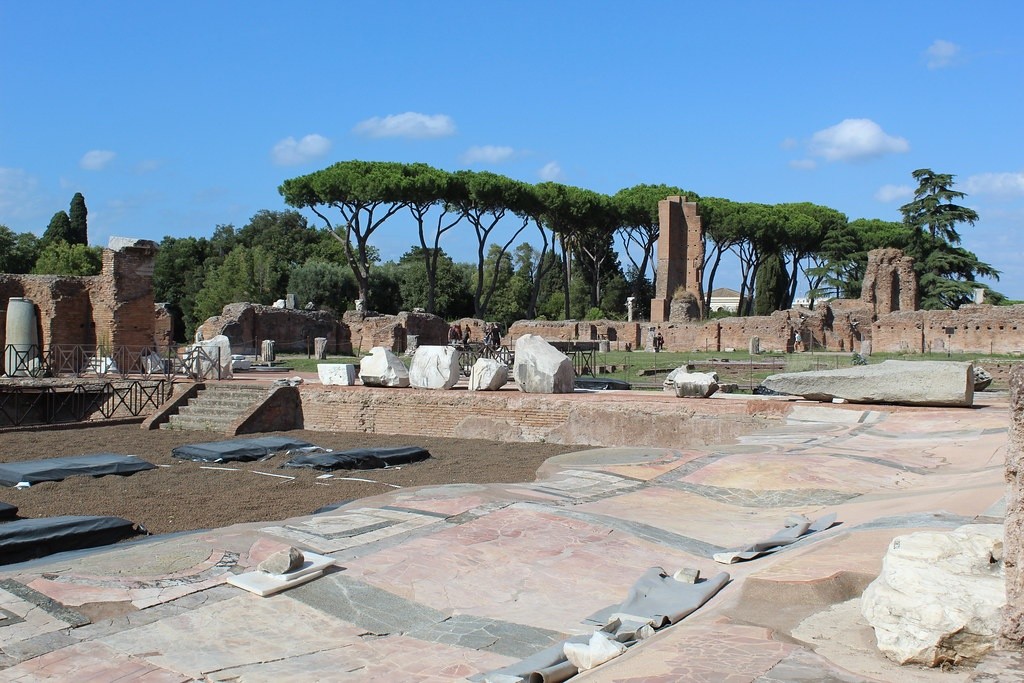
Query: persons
[[448, 321, 500, 352], [793, 330, 802, 352], [657, 333, 664, 350]]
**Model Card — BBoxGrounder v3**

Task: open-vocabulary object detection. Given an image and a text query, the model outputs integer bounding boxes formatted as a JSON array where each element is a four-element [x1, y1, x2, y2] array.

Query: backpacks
[[467, 337, 472, 343], [451, 330, 458, 338]]
